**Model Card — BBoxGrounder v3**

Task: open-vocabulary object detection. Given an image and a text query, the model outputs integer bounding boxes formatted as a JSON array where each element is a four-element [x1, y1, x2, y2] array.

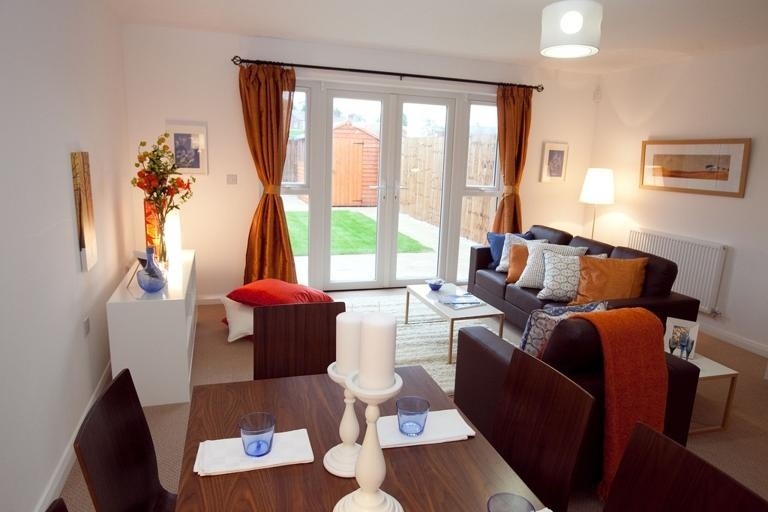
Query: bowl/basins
[[428, 283, 442, 291]]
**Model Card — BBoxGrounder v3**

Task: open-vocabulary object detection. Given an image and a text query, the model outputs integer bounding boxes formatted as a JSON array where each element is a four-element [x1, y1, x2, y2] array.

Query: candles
[[360, 313, 394, 384], [335, 312, 362, 373]]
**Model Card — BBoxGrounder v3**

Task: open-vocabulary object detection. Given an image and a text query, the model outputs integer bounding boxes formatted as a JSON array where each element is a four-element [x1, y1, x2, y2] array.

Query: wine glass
[[669, 331, 694, 362]]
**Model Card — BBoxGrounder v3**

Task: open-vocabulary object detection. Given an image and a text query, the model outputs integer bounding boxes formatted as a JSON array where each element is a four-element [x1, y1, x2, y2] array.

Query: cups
[[238, 411, 276, 457], [396, 396, 431, 435], [486, 491, 537, 512]]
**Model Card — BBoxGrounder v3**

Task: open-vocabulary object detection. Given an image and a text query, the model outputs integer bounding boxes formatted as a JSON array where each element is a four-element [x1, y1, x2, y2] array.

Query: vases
[[144, 195, 166, 257]]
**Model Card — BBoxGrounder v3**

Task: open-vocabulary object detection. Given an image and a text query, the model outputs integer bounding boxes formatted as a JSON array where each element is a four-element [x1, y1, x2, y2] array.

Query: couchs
[[466, 224, 702, 329]]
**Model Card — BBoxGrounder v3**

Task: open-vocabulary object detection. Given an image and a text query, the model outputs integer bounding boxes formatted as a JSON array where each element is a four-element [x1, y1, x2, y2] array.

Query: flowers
[[127, 130, 198, 260]]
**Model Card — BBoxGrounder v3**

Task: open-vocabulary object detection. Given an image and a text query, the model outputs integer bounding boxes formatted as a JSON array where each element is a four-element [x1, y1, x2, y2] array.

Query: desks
[[172, 365, 552, 512]]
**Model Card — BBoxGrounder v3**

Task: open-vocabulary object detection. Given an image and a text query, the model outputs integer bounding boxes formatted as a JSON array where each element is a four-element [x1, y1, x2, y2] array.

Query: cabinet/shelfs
[[104, 249, 197, 410], [676, 352, 738, 436]]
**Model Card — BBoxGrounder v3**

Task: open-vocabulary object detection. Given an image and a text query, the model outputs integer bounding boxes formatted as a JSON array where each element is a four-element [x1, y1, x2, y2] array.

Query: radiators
[[625, 228, 729, 320]]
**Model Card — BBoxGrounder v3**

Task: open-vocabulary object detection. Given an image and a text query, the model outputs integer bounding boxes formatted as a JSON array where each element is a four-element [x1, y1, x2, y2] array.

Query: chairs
[[253, 302, 345, 379], [601, 421, 767, 512], [73, 368, 178, 512], [491, 347, 594, 512], [452, 306, 703, 491]]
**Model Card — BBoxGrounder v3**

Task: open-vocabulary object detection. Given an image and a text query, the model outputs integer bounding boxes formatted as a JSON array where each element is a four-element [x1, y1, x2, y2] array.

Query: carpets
[[335, 293, 521, 398]]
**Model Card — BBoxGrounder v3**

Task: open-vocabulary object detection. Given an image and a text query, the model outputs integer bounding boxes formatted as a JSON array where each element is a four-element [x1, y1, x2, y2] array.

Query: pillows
[[485, 229, 649, 304], [216, 293, 254, 344], [226, 277, 333, 310], [517, 300, 610, 363], [221, 317, 254, 345]]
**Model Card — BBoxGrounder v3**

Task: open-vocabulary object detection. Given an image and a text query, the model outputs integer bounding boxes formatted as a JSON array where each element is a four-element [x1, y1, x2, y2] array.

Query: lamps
[[578, 167, 615, 241], [538, 0, 604, 60]]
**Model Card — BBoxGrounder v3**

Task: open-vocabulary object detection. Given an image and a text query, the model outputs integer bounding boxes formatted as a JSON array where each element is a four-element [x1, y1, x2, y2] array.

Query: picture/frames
[[663, 317, 699, 360], [638, 136, 754, 198], [539, 141, 570, 183], [162, 126, 206, 175]]
